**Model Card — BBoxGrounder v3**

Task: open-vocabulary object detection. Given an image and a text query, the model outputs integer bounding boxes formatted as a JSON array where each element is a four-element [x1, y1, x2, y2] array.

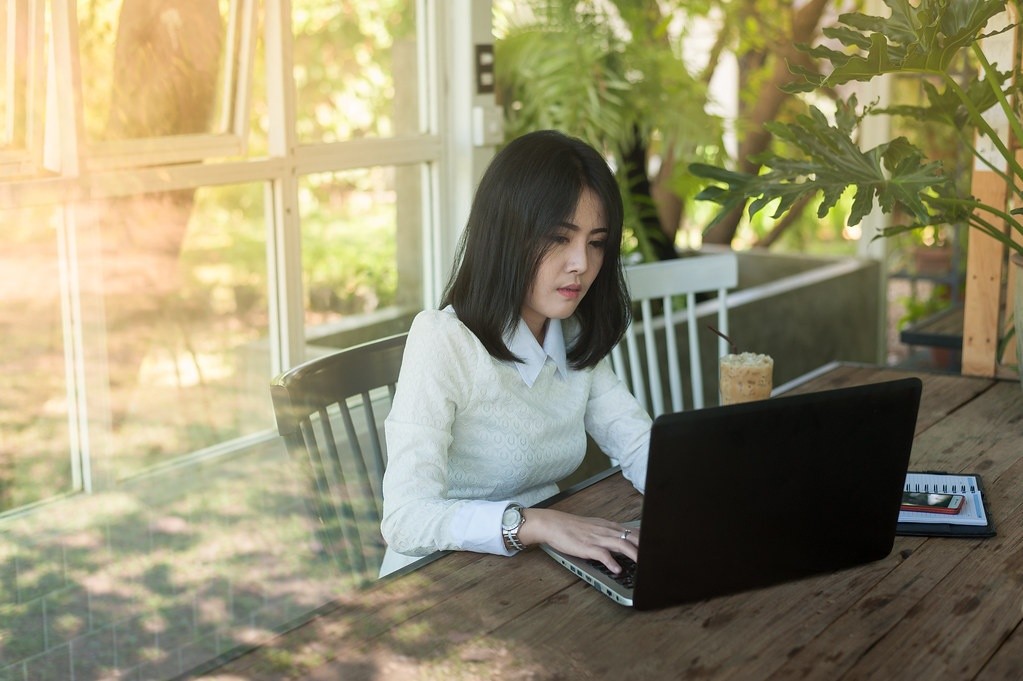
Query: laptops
[[538, 377, 923, 607]]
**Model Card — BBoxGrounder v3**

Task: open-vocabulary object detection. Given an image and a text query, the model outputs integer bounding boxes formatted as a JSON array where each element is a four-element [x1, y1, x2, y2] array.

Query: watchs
[[499, 504, 530, 552]]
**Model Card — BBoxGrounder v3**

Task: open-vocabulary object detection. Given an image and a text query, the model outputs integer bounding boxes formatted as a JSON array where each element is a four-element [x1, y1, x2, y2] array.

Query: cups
[[720, 353, 774, 405]]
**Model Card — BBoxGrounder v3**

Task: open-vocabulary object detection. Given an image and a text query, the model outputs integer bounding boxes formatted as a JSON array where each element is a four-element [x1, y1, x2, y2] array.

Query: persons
[[379, 129, 658, 583]]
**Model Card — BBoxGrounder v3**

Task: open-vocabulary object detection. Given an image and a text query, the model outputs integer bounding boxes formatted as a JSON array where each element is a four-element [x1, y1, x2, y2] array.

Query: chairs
[[604, 251, 742, 468], [268, 329, 412, 593]]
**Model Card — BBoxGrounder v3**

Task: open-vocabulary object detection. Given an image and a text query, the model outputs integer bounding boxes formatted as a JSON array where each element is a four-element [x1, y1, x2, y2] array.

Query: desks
[[901, 298, 1007, 348], [161, 357, 1023, 681]]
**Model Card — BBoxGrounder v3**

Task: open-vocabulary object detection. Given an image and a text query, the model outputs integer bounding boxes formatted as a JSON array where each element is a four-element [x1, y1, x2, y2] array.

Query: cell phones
[[900, 491, 965, 515]]
